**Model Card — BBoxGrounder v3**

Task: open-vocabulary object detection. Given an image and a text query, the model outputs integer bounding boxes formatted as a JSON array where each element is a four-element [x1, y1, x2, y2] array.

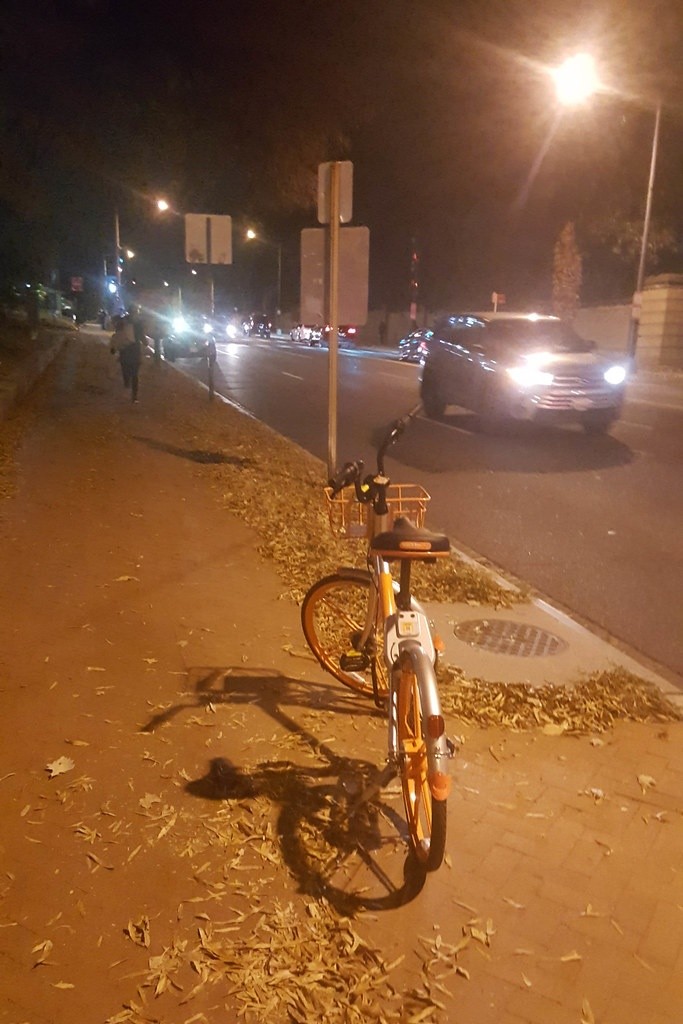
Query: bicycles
[[297, 400, 468, 872]]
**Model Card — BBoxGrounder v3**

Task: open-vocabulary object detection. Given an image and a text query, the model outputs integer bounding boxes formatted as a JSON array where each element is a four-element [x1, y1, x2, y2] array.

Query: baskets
[[323, 484, 432, 539]]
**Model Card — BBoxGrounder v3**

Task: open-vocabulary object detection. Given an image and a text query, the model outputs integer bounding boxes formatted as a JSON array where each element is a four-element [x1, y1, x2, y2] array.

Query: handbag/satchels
[[110, 325, 136, 350]]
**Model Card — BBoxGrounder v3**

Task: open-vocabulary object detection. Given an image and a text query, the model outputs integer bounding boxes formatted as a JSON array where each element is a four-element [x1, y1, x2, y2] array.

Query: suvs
[[244, 310, 273, 340], [420, 311, 632, 438], [320, 325, 358, 349]]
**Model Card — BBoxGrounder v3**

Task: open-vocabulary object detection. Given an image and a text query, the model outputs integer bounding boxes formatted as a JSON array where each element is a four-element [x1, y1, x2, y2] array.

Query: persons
[[110, 304, 148, 403]]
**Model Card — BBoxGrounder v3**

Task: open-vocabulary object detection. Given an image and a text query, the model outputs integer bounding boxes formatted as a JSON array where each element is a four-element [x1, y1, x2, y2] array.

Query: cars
[[122, 298, 238, 362], [290, 323, 316, 342], [398, 329, 426, 362]]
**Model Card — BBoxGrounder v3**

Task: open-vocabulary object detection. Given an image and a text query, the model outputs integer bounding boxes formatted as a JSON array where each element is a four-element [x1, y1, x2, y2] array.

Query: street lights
[[157, 200, 217, 314], [244, 228, 284, 323], [542, 54, 667, 337]]
